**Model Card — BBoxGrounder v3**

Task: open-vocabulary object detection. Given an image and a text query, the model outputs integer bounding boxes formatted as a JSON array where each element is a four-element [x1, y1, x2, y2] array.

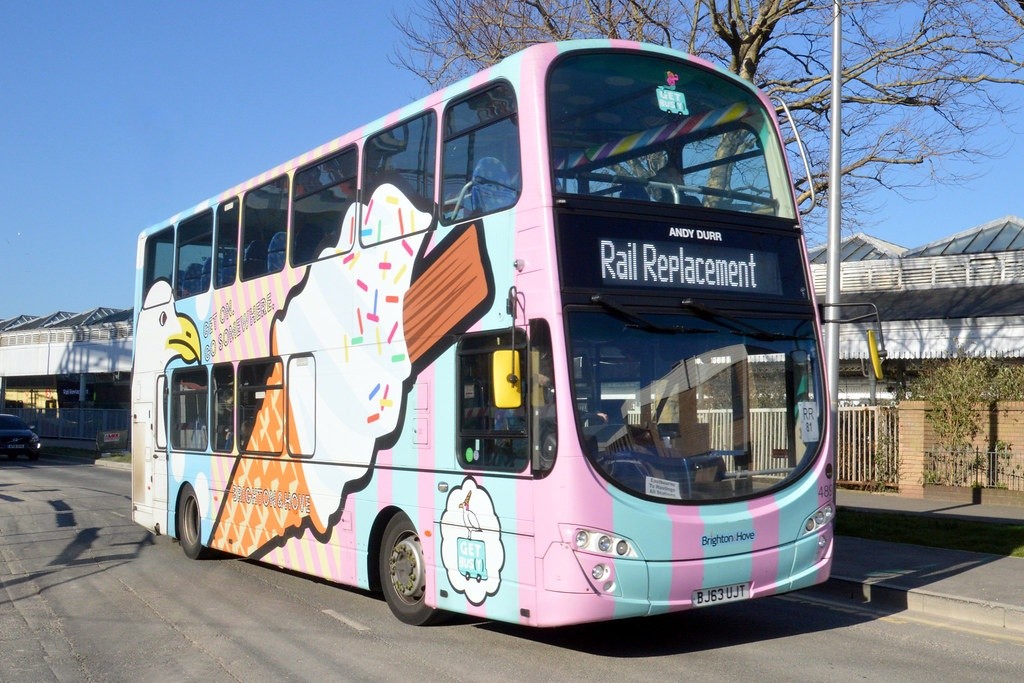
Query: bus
[[129, 37, 889, 627]]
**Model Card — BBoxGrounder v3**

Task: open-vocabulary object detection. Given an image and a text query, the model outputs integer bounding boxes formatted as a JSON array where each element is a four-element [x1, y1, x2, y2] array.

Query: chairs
[[169, 219, 337, 302], [470, 156, 518, 215]]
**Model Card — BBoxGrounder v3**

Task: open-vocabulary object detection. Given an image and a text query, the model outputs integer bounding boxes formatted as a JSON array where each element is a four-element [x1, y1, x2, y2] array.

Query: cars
[[0, 413, 41, 463]]
